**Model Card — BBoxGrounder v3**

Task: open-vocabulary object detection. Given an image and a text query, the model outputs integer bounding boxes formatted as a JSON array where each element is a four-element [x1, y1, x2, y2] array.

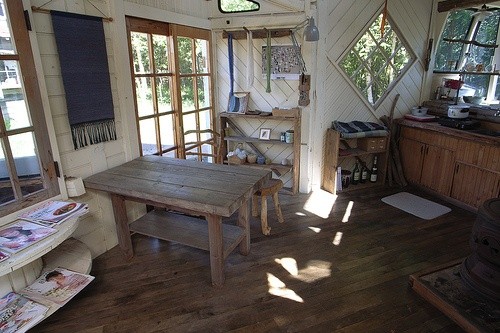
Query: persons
[[46, 271, 90, 292], [0, 226, 50, 248]]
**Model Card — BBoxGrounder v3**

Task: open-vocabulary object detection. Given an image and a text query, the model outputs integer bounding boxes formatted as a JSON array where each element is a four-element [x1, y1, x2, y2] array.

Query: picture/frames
[[226, 92, 249, 115], [259, 128, 271, 141]]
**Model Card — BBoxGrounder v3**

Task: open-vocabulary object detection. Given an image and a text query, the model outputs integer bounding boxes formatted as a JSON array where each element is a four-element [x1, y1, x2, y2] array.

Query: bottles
[[341, 170, 352, 187], [282, 158, 287, 165], [281, 130, 294, 143], [371, 156, 378, 182], [247, 153, 270, 165], [352, 160, 360, 185], [360, 161, 368, 182]]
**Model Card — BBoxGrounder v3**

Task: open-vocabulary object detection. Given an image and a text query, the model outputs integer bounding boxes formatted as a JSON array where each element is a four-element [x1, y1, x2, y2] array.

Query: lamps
[[290, 16, 319, 73]]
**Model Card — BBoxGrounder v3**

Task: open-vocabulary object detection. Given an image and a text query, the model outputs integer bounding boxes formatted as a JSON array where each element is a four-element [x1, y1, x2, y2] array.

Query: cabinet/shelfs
[[393, 118, 500, 214], [217, 109, 301, 195], [323, 128, 391, 196], [0, 199, 92, 333]]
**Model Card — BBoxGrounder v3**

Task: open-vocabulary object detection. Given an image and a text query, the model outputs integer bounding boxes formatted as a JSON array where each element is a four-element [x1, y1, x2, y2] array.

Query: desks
[[83, 154, 272, 289]]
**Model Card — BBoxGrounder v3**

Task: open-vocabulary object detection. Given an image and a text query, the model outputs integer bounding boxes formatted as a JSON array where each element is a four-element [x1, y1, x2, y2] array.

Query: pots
[[448, 104, 471, 119]]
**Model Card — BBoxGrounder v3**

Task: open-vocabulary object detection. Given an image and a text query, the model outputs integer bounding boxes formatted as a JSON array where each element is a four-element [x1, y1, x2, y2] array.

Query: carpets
[[381, 192, 452, 220]]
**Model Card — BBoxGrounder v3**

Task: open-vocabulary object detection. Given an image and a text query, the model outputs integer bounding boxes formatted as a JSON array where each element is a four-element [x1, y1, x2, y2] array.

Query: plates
[[47, 202, 81, 218]]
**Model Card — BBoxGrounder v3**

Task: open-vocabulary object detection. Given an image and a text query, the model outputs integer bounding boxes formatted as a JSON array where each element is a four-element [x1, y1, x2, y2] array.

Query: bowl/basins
[[411, 106, 429, 116], [463, 96, 488, 105], [446, 79, 465, 90]]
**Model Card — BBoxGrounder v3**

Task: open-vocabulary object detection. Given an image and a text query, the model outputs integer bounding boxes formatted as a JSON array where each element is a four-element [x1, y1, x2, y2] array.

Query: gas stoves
[[438, 117, 480, 131]]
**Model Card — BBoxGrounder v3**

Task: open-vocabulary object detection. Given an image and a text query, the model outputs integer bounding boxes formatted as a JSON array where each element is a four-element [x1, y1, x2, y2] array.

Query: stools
[[251, 179, 285, 235]]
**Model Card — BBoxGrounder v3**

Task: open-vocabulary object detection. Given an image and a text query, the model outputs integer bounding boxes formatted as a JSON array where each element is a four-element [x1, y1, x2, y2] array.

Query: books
[[0, 199, 90, 264], [0, 266, 95, 333]]
